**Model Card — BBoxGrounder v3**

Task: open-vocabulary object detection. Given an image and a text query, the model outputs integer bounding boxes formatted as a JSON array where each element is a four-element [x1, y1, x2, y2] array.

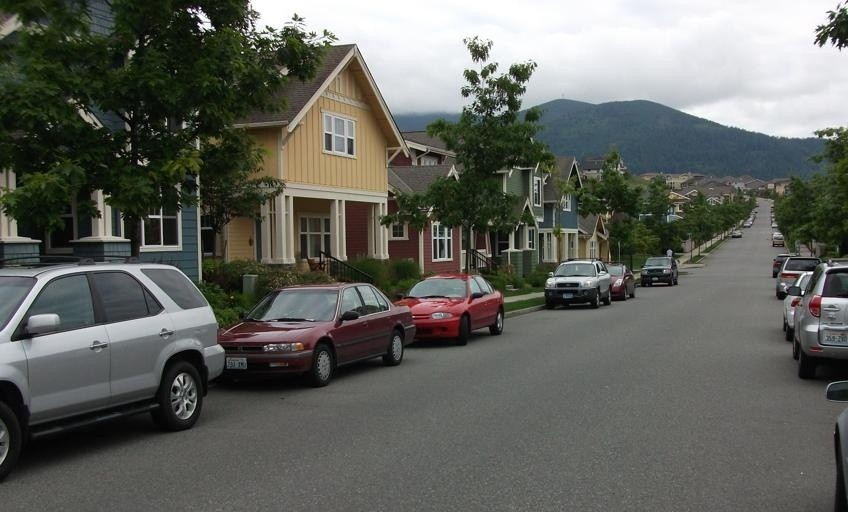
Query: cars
[[215, 281, 415, 393], [767, 203, 847, 511], [391, 274, 514, 347], [540, 253, 679, 314], [729, 199, 758, 240]]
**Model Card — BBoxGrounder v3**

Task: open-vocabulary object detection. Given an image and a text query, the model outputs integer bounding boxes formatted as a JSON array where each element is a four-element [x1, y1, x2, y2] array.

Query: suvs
[[0, 252, 232, 487]]
